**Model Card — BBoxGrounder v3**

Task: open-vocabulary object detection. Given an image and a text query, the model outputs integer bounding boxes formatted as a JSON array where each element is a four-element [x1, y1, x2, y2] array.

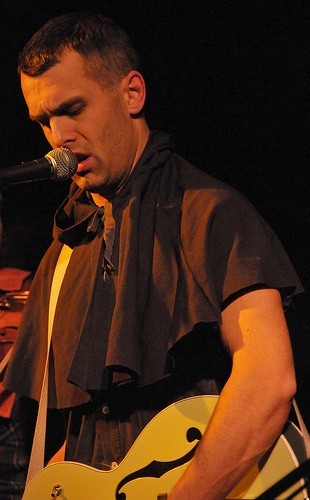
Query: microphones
[[0, 147, 77, 189]]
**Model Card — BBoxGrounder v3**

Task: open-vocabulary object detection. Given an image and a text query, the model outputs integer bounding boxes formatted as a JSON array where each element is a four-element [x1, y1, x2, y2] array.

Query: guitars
[[20, 395, 310, 500]]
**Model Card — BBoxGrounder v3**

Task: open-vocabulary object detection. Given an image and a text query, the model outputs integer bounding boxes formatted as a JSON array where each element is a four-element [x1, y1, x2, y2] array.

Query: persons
[[0, 14, 305, 500]]
